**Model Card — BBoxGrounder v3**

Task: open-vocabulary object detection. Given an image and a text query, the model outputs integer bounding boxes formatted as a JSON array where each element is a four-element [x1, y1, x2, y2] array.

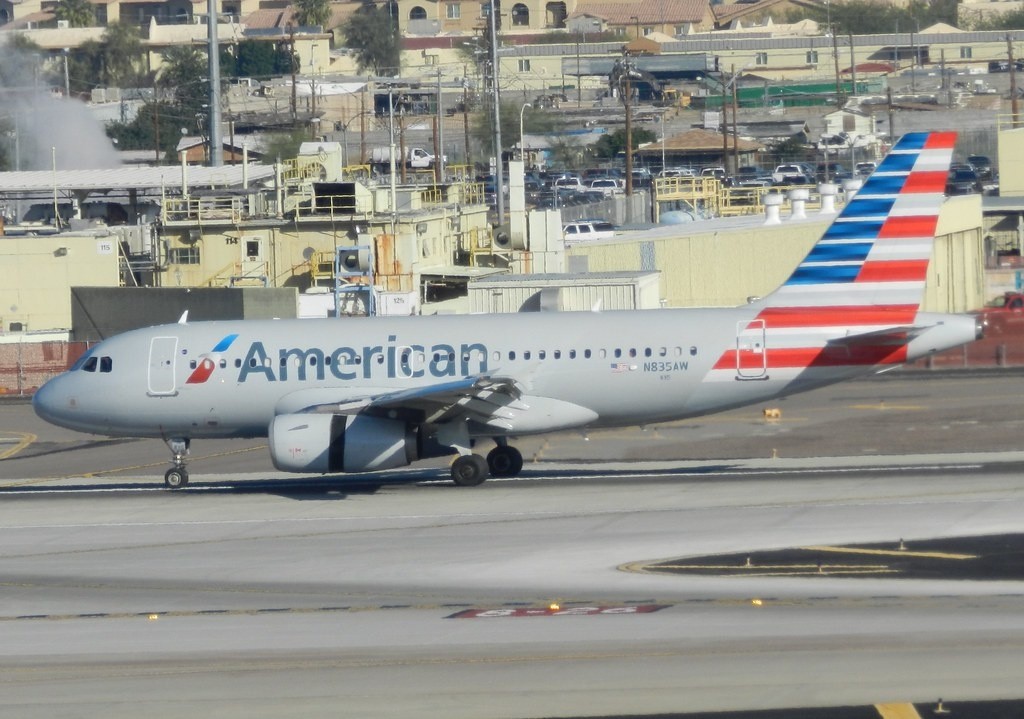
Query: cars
[[563, 220, 617, 240], [738, 181, 770, 188], [986, 61, 1022, 72], [818, 163, 848, 176], [784, 162, 816, 172], [587, 180, 618, 195], [944, 154, 994, 194], [471, 168, 729, 195]]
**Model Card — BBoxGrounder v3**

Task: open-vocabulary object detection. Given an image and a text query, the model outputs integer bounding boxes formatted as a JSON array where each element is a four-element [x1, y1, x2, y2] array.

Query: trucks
[[366, 144, 449, 168], [220, 77, 263, 98], [663, 88, 692, 108]]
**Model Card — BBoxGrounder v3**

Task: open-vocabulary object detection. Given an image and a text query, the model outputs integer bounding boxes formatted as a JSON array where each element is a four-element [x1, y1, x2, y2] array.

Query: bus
[[375, 87, 440, 115]]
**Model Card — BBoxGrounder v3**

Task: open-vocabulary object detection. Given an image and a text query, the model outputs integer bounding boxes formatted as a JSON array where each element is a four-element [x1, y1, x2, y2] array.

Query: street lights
[[630, 16, 639, 39], [329, 83, 365, 164], [519, 98, 532, 160], [716, 52, 764, 177], [310, 111, 373, 164], [309, 43, 319, 115], [462, 42, 495, 138]]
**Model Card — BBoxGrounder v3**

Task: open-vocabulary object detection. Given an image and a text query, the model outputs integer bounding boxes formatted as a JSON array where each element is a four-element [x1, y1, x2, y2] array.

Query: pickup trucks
[[31, 132, 984, 497], [854, 161, 878, 176], [772, 164, 808, 185], [550, 177, 583, 190]]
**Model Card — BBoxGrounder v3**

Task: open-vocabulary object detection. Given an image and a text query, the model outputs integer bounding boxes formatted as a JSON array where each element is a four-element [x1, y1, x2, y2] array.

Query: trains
[[18, 199, 172, 224]]
